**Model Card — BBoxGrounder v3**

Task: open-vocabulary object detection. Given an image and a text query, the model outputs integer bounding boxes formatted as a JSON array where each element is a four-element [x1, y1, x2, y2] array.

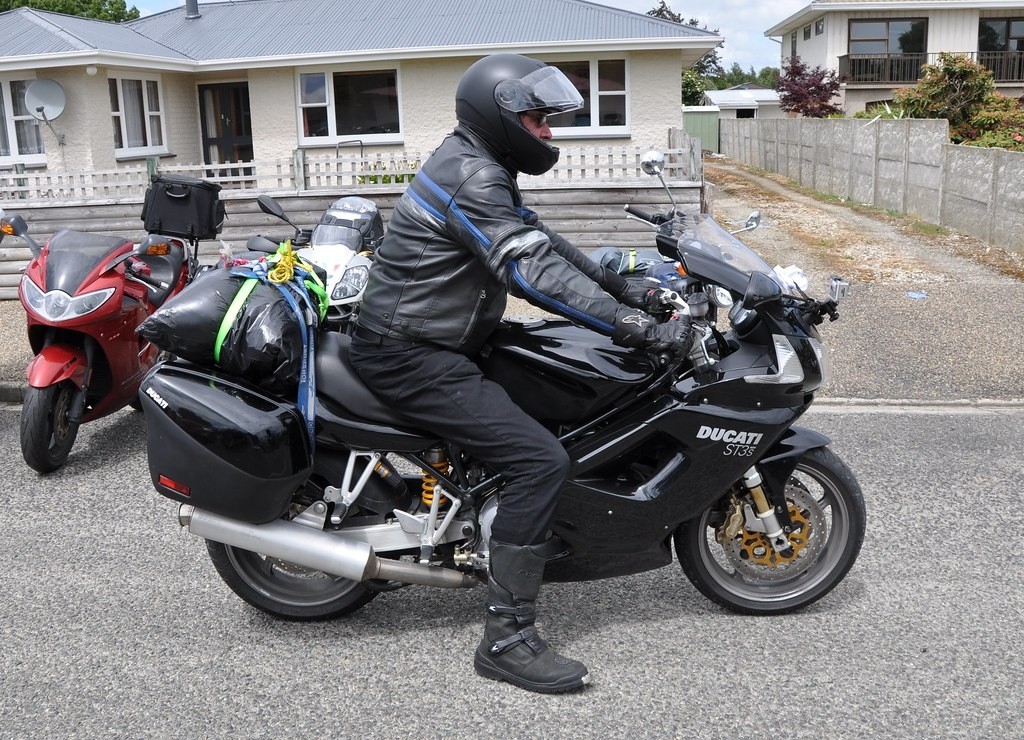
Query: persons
[[348, 53, 694, 691]]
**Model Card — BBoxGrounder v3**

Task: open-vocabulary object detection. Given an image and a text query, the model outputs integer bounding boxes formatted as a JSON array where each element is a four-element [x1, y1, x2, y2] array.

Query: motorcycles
[[138, 149, 869, 624], [1, 171, 228, 475], [247, 192, 388, 254]]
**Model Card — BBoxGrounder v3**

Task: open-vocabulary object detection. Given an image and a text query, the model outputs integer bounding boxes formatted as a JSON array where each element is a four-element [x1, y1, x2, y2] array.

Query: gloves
[[600, 266, 661, 311], [612, 304, 694, 362]]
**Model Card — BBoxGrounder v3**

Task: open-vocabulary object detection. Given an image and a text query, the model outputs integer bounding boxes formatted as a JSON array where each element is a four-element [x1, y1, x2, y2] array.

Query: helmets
[[456, 54, 564, 176]]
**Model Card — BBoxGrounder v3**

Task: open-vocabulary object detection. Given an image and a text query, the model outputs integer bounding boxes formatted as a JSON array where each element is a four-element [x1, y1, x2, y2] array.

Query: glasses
[[523, 112, 549, 128]]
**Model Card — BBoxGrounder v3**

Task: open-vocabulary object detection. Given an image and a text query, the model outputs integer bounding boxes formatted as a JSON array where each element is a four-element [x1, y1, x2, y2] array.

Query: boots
[[474, 535, 590, 691]]
